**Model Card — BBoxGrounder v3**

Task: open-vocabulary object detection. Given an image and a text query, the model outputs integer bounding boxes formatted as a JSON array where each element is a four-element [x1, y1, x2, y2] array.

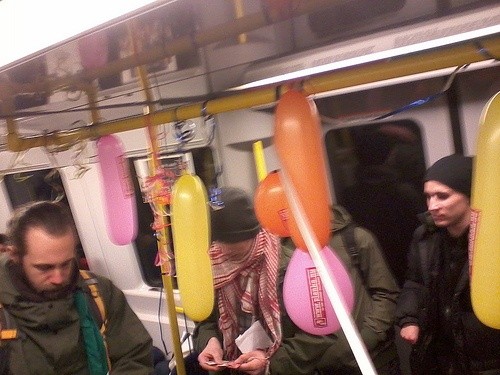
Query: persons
[[395, 154, 500, 375], [1, 200, 154, 375], [269, 206, 401, 375], [171, 187, 294, 375]]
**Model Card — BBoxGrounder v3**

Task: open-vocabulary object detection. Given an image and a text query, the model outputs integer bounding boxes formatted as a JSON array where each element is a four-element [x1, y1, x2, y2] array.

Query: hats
[[203, 186, 259, 243], [424, 155, 472, 196]]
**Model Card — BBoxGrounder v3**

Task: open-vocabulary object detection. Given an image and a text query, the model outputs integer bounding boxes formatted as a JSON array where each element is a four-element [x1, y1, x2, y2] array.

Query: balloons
[[79, 31, 109, 69], [276, 80, 331, 253], [283, 245, 354, 335], [255, 169, 292, 237], [468, 91, 500, 330], [97, 135, 137, 245], [171, 172, 215, 321]]
